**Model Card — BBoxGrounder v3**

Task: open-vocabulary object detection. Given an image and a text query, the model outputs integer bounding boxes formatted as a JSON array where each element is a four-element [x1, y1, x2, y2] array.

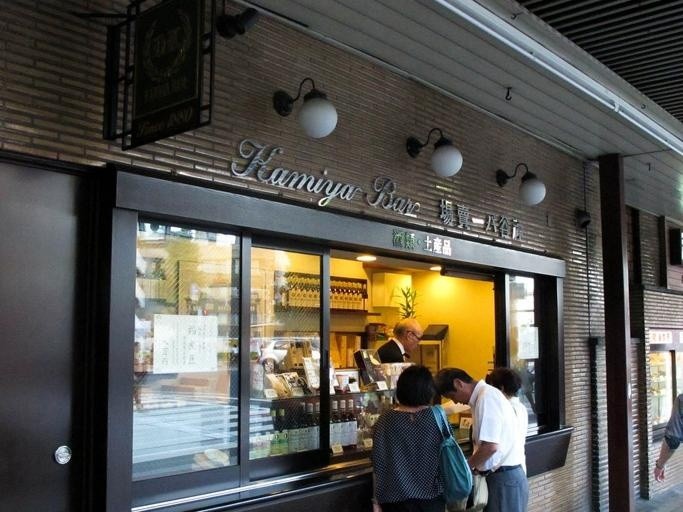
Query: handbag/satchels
[[432, 404, 472, 512], [468, 474, 488, 512]]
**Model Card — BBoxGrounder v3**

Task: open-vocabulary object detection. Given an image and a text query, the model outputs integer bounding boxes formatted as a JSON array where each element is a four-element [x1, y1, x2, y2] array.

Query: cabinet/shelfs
[[368, 340, 442, 375]]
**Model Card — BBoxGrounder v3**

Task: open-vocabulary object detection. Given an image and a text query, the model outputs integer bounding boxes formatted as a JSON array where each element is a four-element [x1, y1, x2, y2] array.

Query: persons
[[370, 366, 457, 511], [434, 367, 529, 511], [132, 342, 148, 410], [653, 394, 683, 484], [486, 368, 528, 476], [372, 318, 423, 361]]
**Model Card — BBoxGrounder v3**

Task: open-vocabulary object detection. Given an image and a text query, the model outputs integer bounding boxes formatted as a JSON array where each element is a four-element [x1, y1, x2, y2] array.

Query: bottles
[[277, 276, 369, 312], [249, 392, 393, 460]]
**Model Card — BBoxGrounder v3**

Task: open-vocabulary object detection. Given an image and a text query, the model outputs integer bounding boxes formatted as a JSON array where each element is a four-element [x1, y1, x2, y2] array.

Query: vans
[[257, 338, 320, 372]]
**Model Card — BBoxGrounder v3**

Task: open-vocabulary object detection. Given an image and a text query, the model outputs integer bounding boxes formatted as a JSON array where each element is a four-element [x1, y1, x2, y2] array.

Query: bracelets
[[467, 462, 476, 471], [656, 461, 664, 470]]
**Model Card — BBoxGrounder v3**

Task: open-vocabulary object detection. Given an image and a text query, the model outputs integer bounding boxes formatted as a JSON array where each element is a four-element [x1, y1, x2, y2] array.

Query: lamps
[[406, 127, 463, 178], [272, 77, 338, 139], [496, 162, 546, 207]]
